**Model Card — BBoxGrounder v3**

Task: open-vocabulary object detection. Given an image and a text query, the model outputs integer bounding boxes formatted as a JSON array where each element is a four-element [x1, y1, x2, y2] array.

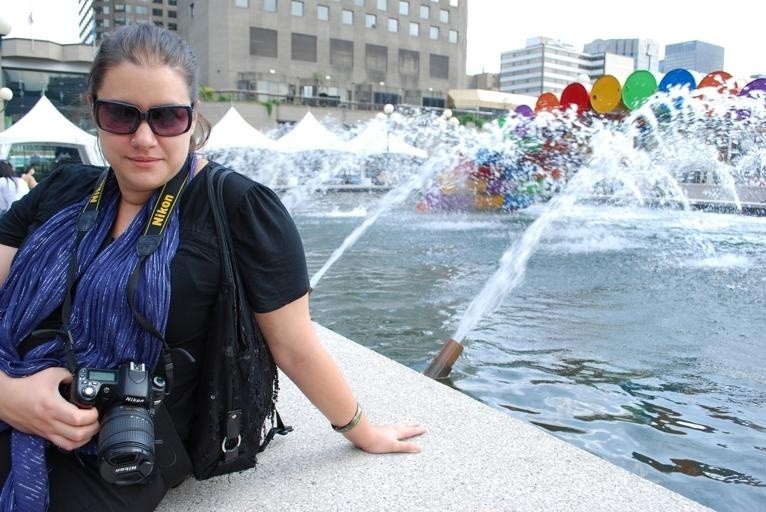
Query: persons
[[0, 161, 108, 290], [0, 18, 428, 509], [20, 163, 40, 192], [1, 159, 32, 225]]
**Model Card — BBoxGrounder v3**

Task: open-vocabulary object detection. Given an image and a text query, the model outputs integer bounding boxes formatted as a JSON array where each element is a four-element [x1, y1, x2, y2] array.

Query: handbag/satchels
[[178, 162, 281, 484]]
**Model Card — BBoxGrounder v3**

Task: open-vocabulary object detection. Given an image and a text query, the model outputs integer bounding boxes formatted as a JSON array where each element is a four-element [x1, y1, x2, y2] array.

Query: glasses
[[90, 93, 196, 136]]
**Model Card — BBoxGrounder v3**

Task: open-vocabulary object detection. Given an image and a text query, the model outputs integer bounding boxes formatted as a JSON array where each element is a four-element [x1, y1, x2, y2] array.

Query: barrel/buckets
[[413, 67, 766, 215]]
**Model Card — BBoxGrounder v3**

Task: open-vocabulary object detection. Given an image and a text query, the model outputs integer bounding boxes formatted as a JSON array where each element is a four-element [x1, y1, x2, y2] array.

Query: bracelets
[[330, 402, 363, 434]]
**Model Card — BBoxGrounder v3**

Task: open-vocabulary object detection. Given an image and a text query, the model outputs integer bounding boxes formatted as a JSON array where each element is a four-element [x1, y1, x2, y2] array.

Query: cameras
[[67, 358, 168, 487]]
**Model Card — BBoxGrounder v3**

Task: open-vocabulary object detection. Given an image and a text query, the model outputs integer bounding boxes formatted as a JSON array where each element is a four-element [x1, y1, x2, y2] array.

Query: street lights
[[384, 104, 395, 153]]
